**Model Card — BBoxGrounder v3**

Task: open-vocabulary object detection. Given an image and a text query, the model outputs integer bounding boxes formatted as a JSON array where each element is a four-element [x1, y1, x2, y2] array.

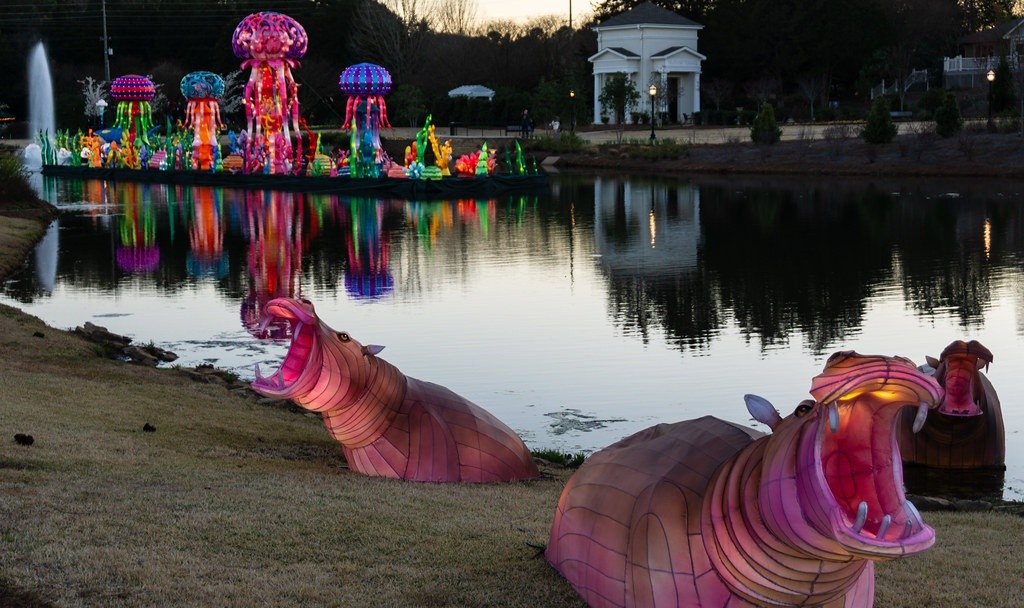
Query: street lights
[[568, 88, 576, 135], [985, 69, 997, 128], [649, 85, 658, 141]]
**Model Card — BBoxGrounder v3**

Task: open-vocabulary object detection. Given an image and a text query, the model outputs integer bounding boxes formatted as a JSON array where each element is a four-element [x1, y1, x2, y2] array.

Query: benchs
[[505, 125, 534, 136]]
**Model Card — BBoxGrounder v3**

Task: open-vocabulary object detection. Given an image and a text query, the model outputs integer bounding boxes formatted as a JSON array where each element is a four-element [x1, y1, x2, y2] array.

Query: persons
[[521, 109, 535, 138], [549, 116, 560, 131]]
